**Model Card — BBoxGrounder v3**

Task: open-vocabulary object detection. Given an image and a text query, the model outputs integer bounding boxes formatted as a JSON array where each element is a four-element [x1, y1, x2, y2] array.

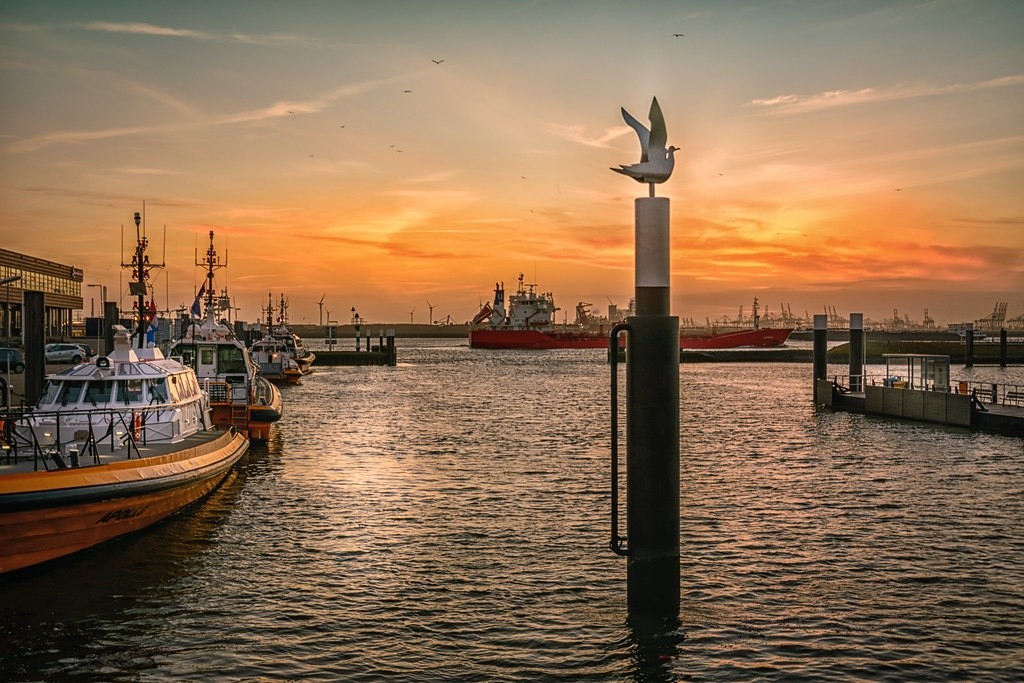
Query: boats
[[0, 202, 315, 576], [467, 300, 791, 350]]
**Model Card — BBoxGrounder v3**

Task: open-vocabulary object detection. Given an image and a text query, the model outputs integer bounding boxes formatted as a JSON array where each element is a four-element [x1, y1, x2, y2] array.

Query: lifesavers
[[133, 412, 141, 442]]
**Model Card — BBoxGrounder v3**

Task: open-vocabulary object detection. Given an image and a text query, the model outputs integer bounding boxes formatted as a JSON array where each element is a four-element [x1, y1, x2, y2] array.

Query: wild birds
[[529, 209, 534, 213], [802, 234, 807, 236], [718, 173, 723, 175], [308, 154, 314, 157], [432, 59, 445, 65], [776, 232, 780, 235], [521, 176, 527, 180], [286, 111, 294, 114], [402, 90, 413, 93], [609, 96, 680, 184], [390, 145, 395, 148], [894, 189, 904, 191], [341, 125, 345, 129], [395, 149, 403, 152]]
